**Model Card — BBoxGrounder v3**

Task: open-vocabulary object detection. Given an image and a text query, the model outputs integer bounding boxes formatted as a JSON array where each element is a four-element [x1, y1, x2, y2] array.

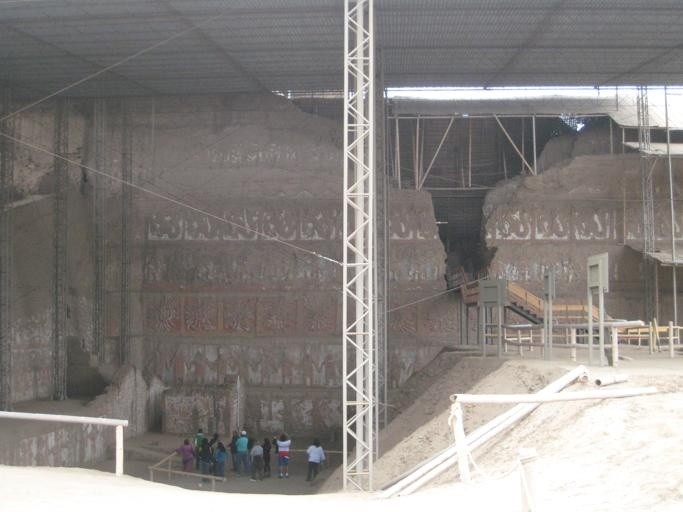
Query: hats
[[241, 430, 247, 436]]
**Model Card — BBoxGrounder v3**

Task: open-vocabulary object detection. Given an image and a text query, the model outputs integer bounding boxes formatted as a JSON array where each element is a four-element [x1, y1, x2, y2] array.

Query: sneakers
[[306, 477, 314, 481], [231, 467, 270, 481], [280, 472, 288, 477]]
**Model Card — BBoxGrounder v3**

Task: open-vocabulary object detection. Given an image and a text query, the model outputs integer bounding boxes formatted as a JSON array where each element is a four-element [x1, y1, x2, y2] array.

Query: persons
[[175, 428, 292, 483], [305, 436, 326, 481]]
